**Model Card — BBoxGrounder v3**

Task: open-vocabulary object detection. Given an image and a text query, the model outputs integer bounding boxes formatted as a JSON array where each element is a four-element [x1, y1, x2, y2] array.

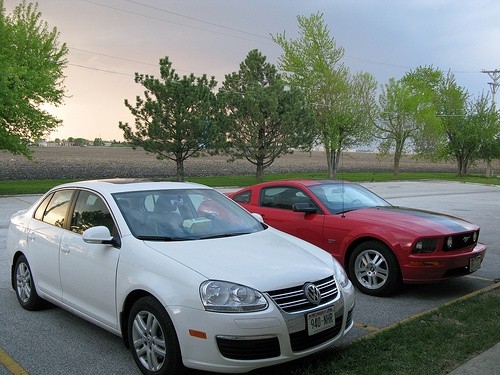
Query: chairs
[[94, 195, 183, 233], [274, 188, 328, 207]]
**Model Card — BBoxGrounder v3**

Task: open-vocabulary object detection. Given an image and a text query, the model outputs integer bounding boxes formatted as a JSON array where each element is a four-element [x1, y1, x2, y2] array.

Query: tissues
[[182, 217, 212, 234]]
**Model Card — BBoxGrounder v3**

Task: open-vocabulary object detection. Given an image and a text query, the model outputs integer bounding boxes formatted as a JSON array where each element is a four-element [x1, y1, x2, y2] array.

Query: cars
[[197, 177, 487, 297], [6, 178, 355, 375]]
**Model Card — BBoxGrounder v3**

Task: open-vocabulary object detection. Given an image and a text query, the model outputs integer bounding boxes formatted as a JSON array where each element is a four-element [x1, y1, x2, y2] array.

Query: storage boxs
[[183, 218, 212, 234]]
